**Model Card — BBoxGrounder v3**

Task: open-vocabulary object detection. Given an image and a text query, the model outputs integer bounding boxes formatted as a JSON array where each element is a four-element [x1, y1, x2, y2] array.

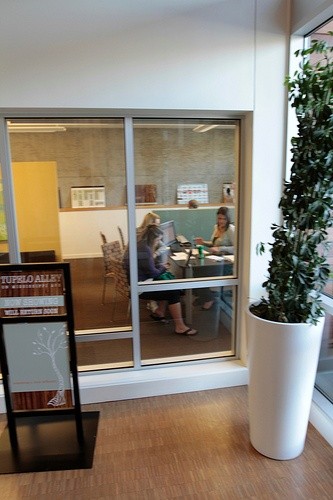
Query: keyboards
[[171, 243, 186, 251]]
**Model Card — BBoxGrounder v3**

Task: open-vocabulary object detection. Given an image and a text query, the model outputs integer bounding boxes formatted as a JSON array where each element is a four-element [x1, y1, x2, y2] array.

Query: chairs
[[100, 226, 130, 323]]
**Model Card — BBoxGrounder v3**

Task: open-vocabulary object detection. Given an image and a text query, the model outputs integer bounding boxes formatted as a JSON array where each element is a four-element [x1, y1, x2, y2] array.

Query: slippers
[[150, 313, 168, 323], [175, 327, 198, 336]]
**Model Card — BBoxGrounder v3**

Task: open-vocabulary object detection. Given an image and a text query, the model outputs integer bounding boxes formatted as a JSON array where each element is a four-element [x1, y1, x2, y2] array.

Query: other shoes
[[147, 303, 158, 311], [200, 300, 215, 311]]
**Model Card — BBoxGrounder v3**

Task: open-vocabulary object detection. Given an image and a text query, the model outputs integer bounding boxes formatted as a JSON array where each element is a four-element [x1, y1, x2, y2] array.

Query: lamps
[[193, 124, 217, 133], [7, 127, 67, 133]]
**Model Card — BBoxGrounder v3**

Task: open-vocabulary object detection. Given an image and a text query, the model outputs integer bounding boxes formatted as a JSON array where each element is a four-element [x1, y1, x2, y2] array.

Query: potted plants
[[247, 28, 333, 461]]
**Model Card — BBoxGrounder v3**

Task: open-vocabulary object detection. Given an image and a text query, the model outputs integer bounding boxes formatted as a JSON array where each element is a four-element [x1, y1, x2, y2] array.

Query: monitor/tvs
[[158, 220, 178, 247]]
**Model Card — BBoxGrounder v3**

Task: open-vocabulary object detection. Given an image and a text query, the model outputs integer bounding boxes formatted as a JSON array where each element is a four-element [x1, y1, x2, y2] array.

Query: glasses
[[218, 216, 227, 220]]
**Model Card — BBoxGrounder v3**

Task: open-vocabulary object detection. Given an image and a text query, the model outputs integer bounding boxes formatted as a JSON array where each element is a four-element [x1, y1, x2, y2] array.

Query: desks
[[166, 248, 234, 325]]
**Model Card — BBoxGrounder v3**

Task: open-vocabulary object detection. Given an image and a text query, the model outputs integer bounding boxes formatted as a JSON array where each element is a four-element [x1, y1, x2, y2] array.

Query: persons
[[122, 212, 160, 312], [224, 188, 233, 203], [192, 207, 235, 311], [188, 200, 198, 209], [127, 226, 199, 336]]
